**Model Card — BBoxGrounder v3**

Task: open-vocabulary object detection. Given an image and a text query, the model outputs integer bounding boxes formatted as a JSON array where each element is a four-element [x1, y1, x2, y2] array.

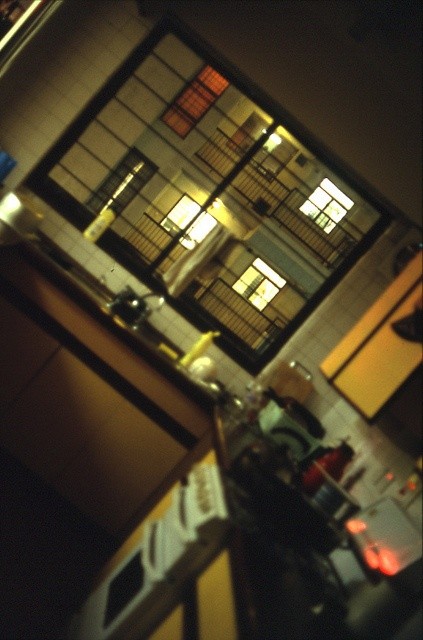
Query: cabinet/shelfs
[[0, 288, 200, 550], [317, 249, 423, 460]]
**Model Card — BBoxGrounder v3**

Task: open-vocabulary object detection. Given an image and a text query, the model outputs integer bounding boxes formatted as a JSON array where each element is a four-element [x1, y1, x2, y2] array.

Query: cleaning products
[[179, 329, 223, 370]]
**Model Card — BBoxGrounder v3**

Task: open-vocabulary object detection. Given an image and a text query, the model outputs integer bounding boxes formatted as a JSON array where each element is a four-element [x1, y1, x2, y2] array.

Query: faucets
[[141, 290, 169, 316]]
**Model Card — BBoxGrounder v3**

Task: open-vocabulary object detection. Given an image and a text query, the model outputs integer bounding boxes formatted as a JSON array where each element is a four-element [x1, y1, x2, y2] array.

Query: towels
[[160, 221, 238, 299]]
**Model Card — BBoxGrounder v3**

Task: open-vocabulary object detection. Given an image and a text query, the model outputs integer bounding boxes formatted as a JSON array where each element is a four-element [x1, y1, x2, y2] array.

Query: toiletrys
[[80, 206, 116, 244]]
